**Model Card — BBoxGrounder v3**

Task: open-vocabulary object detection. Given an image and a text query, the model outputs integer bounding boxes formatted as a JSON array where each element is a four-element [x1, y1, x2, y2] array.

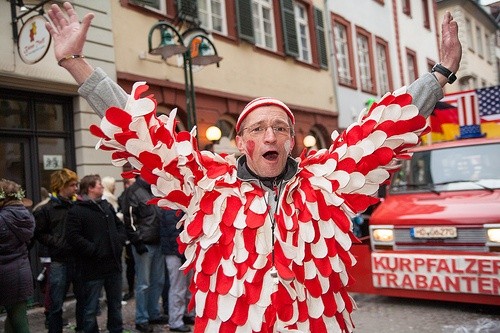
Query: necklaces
[[257, 178, 283, 277]]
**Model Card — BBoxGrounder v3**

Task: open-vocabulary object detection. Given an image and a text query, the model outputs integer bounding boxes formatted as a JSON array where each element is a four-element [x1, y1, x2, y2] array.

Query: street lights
[[146, 20, 223, 161]]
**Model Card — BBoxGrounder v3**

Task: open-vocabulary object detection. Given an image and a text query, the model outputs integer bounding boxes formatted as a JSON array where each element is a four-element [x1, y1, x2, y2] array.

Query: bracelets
[[57, 54, 84, 67]]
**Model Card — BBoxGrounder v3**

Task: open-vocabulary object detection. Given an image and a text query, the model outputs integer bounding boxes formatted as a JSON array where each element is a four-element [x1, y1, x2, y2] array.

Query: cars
[[368, 135, 500, 307]]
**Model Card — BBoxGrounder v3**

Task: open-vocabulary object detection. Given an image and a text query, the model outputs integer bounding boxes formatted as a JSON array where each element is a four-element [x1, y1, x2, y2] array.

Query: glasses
[[238, 123, 292, 136]]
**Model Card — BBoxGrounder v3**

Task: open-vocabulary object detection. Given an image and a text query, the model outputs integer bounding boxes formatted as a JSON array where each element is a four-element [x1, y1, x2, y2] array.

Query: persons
[[35, 167, 197, 333], [0, 179, 36, 333], [43, 2, 462, 333]]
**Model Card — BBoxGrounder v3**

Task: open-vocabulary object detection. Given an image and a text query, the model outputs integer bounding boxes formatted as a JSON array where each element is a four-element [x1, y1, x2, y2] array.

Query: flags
[[420, 87, 500, 144]]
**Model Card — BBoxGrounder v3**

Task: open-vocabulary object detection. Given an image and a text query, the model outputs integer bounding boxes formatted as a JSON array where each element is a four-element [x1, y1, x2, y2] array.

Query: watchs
[[432, 62, 457, 85]]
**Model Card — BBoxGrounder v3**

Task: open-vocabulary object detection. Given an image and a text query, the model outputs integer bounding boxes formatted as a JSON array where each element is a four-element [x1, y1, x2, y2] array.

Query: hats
[[235, 97, 295, 134]]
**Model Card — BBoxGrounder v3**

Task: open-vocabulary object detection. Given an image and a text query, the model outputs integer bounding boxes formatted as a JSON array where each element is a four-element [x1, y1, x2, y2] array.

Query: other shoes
[[148, 315, 168, 324], [123, 292, 133, 301], [182, 315, 195, 325], [136, 322, 153, 333], [169, 325, 189, 333]]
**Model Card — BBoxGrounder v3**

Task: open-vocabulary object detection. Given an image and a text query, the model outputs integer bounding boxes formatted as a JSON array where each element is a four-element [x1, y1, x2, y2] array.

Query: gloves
[[135, 245, 148, 255]]
